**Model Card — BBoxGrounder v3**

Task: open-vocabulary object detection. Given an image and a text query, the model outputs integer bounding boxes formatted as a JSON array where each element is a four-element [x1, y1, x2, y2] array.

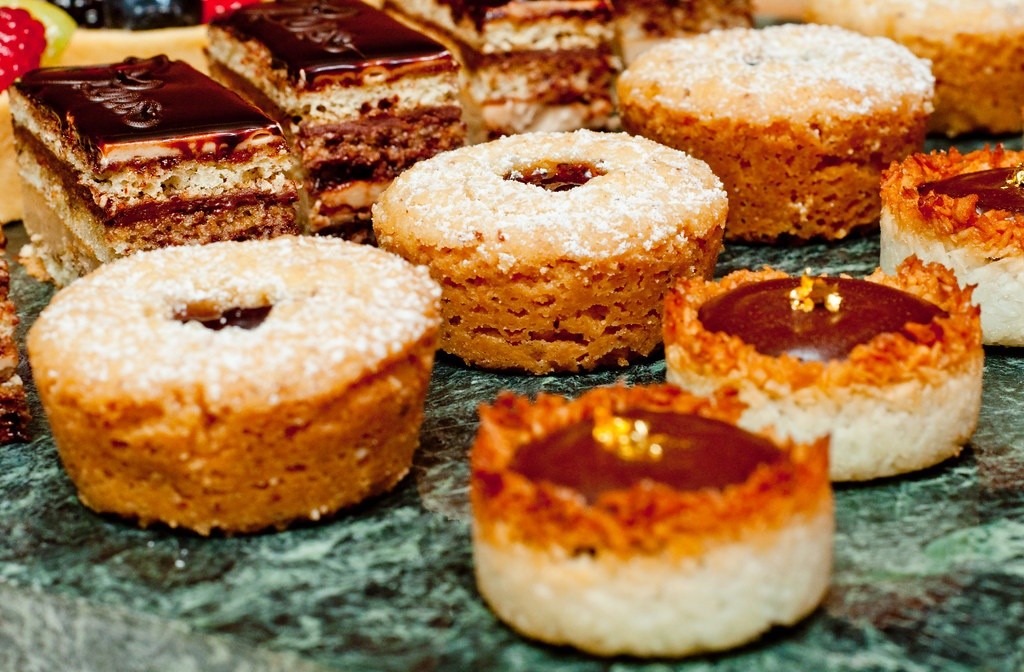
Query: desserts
[[0, 0, 1024, 657]]
